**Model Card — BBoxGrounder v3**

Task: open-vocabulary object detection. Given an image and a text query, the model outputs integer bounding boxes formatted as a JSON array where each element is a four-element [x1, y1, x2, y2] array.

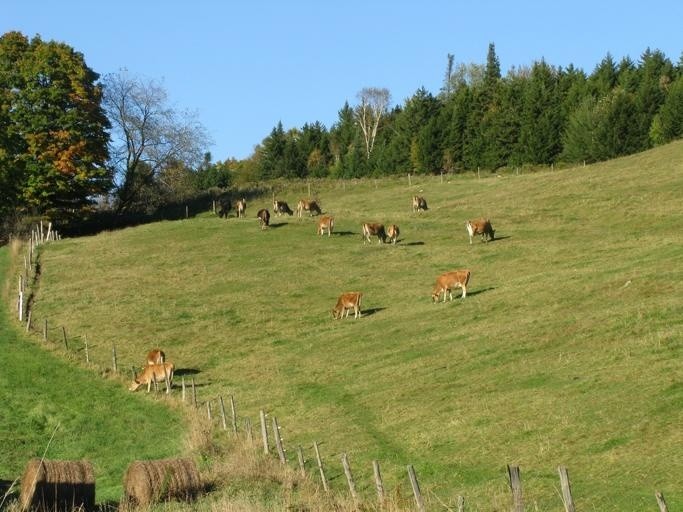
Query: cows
[[255, 208, 271, 229], [127, 347, 175, 393], [430, 269, 471, 304], [411, 195, 430, 213], [465, 217, 497, 245], [361, 221, 400, 245], [331, 291, 364, 320], [316, 216, 336, 237], [218, 197, 250, 219], [272, 199, 322, 218]]
